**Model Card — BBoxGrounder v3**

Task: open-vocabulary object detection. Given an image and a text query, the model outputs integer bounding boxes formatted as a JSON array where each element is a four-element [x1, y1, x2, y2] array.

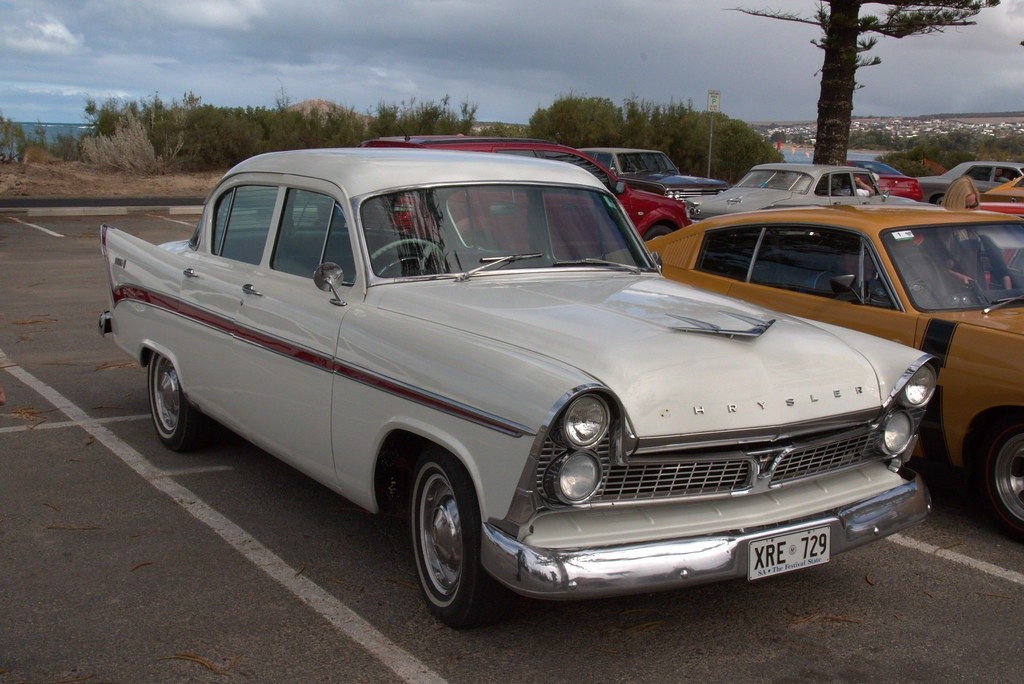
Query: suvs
[[317, 131, 693, 263]]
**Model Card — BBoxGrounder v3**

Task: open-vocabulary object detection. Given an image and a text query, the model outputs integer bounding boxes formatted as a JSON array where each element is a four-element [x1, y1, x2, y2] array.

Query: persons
[[926, 175, 1013, 290], [832, 174, 875, 197]]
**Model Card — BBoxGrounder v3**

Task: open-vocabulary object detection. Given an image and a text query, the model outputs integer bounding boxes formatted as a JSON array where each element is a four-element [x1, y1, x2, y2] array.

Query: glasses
[[966, 199, 979, 208]]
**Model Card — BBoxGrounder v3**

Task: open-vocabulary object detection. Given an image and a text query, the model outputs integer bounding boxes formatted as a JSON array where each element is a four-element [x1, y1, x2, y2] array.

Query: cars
[[908, 199, 1024, 286], [985, 172, 1024, 196], [845, 161, 924, 204], [572, 145, 730, 199], [919, 161, 1024, 208], [103, 151, 944, 629], [688, 163, 924, 246], [601, 205, 1024, 539]]
